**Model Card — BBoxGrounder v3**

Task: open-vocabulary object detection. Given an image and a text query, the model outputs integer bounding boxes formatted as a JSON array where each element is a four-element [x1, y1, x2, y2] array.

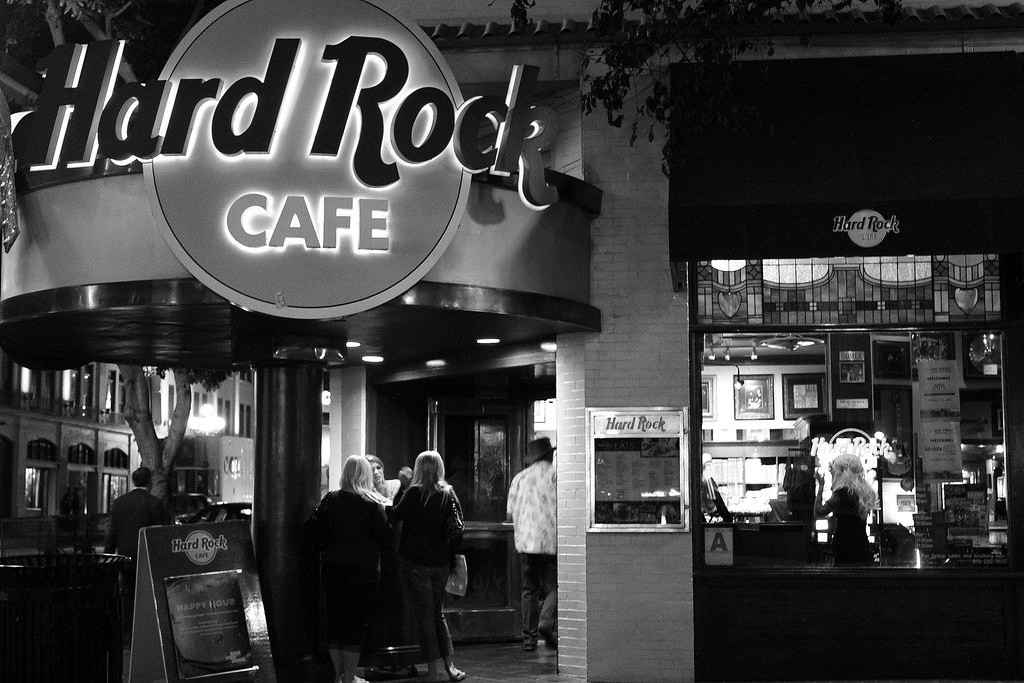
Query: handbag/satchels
[[445, 486, 464, 542]]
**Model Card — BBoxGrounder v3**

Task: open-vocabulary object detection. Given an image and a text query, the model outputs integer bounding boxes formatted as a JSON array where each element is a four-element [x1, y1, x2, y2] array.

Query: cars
[[97, 492, 212, 538], [180, 502, 252, 526]]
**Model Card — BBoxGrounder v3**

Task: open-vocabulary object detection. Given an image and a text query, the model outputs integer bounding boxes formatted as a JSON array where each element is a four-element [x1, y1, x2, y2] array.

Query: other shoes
[[524, 644, 537, 650], [336, 674, 369, 683], [420, 672, 438, 683], [539, 622, 558, 649], [450, 669, 466, 682]]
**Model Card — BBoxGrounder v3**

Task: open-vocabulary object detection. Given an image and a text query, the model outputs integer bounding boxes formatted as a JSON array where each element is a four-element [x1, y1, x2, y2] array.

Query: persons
[[104, 467, 168, 652], [814, 454, 877, 567], [315, 450, 466, 683], [702, 453, 718, 523], [507, 437, 557, 651]]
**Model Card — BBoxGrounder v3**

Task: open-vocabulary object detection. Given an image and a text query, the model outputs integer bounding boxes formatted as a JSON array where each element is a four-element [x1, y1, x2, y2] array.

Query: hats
[[522, 437, 556, 465]]
